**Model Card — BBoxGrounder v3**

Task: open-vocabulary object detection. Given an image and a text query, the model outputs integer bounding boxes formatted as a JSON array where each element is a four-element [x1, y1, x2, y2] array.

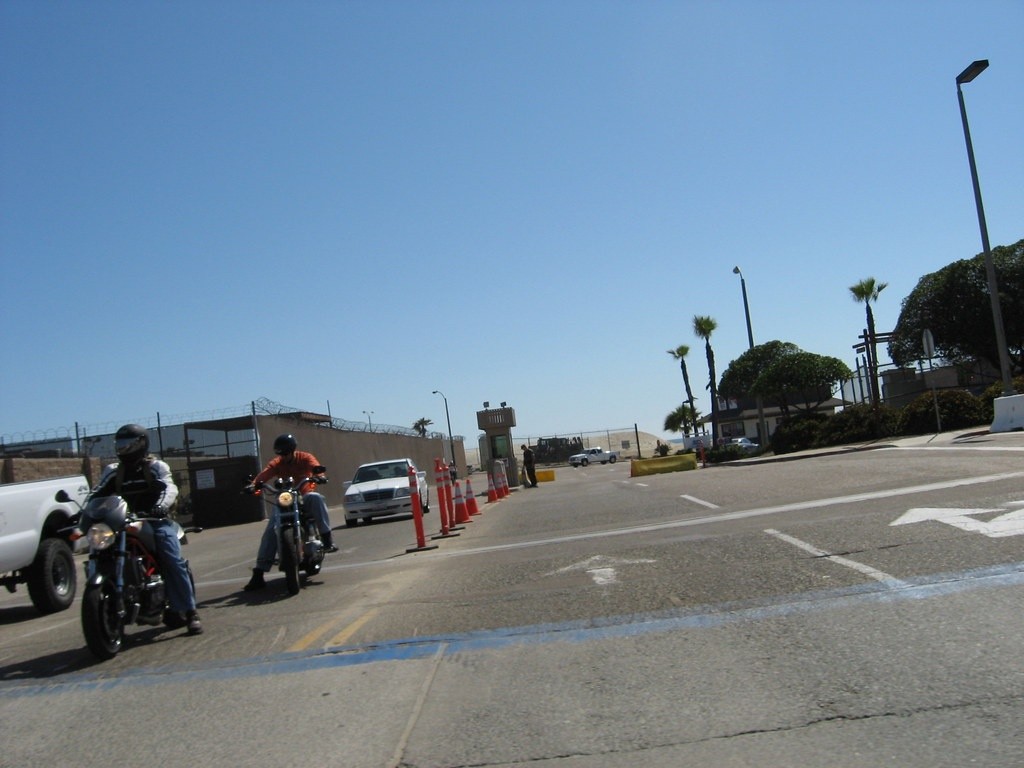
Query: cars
[[342, 458, 429, 527], [721, 437, 760, 451]]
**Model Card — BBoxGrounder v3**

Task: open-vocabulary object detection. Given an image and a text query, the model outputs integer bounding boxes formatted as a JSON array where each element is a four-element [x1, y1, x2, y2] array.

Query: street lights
[[954, 58, 1014, 397], [433, 391, 458, 474], [734, 266, 768, 449]]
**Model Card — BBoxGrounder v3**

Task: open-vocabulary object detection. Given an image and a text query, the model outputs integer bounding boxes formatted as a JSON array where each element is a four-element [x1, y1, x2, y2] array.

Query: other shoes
[[186, 610, 203, 634]]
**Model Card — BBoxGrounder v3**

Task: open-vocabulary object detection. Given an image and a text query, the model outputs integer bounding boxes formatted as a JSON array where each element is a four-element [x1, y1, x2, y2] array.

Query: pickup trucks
[[0, 471, 91, 612], [568, 447, 620, 468]]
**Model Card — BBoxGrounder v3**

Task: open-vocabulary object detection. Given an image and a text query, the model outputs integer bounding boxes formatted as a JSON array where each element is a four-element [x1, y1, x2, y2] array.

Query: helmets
[[114, 423, 150, 463], [273, 434, 297, 455]]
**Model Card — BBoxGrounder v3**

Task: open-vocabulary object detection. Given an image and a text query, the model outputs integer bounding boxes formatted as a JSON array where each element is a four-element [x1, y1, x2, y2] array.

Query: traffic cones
[[496, 473, 512, 499], [464, 479, 483, 515], [452, 482, 472, 523], [485, 474, 500, 503]]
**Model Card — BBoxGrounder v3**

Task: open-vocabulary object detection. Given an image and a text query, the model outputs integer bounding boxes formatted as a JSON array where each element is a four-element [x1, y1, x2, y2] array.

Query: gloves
[[151, 505, 167, 518], [316, 475, 328, 485], [243, 485, 256, 495]]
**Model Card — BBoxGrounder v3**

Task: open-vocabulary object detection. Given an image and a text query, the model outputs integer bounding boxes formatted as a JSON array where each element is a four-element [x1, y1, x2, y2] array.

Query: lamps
[[484, 401, 489, 409], [501, 402, 506, 408]]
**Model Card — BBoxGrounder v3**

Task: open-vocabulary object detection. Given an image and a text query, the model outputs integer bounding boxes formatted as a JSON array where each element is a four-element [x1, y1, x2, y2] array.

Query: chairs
[[367, 470, 380, 480]]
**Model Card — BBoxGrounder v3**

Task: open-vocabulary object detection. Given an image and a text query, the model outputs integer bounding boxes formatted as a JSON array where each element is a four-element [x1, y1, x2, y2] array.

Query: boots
[[243, 568, 265, 591], [321, 531, 339, 552]]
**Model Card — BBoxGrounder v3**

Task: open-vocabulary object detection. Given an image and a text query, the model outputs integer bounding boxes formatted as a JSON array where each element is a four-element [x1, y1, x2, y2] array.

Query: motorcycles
[[53, 490, 206, 657], [241, 466, 327, 597]]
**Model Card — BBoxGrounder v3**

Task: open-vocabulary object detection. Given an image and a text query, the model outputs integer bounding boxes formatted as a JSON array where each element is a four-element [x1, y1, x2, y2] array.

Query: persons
[[449, 461, 457, 480], [521, 445, 537, 487], [394, 467, 405, 476], [244, 435, 339, 592], [69, 424, 202, 635]]
